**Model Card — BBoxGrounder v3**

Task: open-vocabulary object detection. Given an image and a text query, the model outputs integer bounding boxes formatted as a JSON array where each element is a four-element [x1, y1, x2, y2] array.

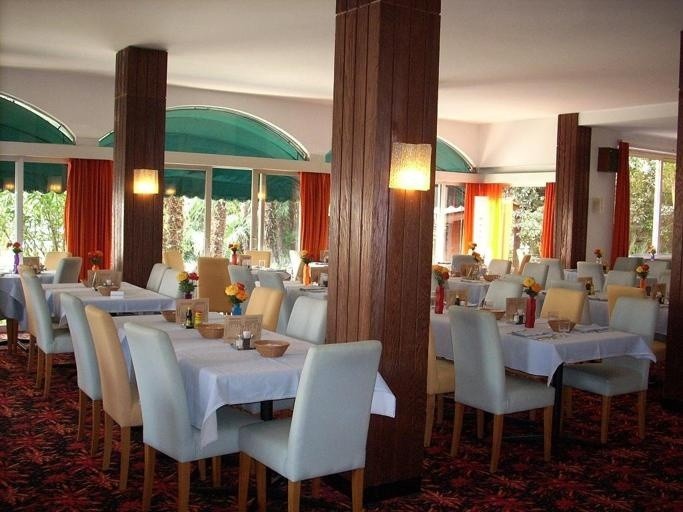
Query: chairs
[[577, 260, 604, 294], [538, 259, 564, 284], [85, 304, 223, 493], [198, 258, 234, 317], [286, 297, 330, 345], [238, 338, 383, 512], [613, 257, 642, 273], [605, 269, 636, 295], [487, 259, 511, 278], [257, 270, 288, 331], [451, 255, 476, 276], [123, 322, 266, 512], [539, 288, 586, 327], [146, 263, 170, 292], [158, 269, 186, 310], [521, 262, 548, 289], [448, 304, 554, 473], [647, 260, 669, 280], [59, 294, 101, 458], [18, 270, 74, 401], [481, 279, 522, 316], [244, 286, 282, 331], [228, 264, 256, 315], [562, 296, 659, 444], [423, 315, 487, 449], [517, 255, 532, 279]]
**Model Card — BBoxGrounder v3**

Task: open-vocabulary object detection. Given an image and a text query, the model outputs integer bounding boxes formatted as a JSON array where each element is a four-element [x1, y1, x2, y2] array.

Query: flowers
[[87, 251, 105, 264], [228, 243, 241, 252], [637, 266, 649, 278], [301, 250, 313, 264], [226, 282, 247, 305], [594, 250, 604, 262], [12, 242, 22, 253], [523, 277, 540, 298], [430, 263, 450, 285], [177, 271, 199, 292]]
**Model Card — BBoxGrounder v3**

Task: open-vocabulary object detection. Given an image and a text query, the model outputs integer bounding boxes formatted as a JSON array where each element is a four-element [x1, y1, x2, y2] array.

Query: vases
[[525, 300, 537, 329], [231, 254, 238, 265], [639, 279, 647, 290], [13, 254, 21, 274], [229, 305, 245, 316], [183, 292, 195, 300], [302, 265, 311, 286], [433, 286, 446, 315], [91, 265, 100, 273]]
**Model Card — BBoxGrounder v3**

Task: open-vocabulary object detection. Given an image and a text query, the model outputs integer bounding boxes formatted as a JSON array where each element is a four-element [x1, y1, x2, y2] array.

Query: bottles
[[322, 273, 328, 287], [455, 296, 459, 306], [242, 331, 250, 349], [516, 309, 523, 324], [586, 278, 591, 294], [185, 306, 193, 328], [602, 263, 607, 274]]
